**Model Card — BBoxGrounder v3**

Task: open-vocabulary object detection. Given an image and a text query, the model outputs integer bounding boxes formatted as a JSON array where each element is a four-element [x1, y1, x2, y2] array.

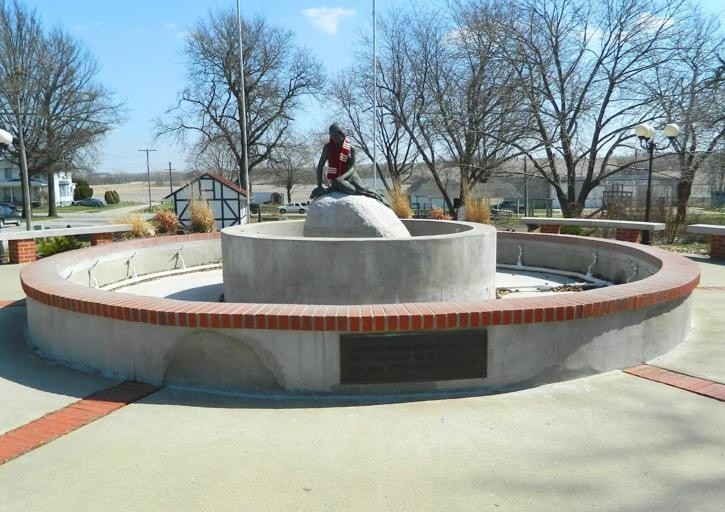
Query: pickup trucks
[[491, 200, 529, 214]]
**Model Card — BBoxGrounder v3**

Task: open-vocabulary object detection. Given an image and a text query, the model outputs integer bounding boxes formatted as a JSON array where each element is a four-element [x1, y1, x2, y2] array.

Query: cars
[[0, 199, 40, 228], [280, 201, 310, 214], [73, 198, 103, 207]]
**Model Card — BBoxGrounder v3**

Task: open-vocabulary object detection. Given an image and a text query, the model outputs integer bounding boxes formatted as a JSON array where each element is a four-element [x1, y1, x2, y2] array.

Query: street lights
[[635, 123, 680, 244]]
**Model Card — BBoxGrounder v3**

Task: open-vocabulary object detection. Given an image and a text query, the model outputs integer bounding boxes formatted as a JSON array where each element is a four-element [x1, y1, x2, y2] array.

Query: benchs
[[521, 217, 667, 244], [687, 224, 725, 261], [0, 224, 133, 264]]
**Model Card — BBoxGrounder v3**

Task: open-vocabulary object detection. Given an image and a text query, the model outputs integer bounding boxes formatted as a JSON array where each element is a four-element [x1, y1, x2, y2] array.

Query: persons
[[309, 122, 392, 207]]
